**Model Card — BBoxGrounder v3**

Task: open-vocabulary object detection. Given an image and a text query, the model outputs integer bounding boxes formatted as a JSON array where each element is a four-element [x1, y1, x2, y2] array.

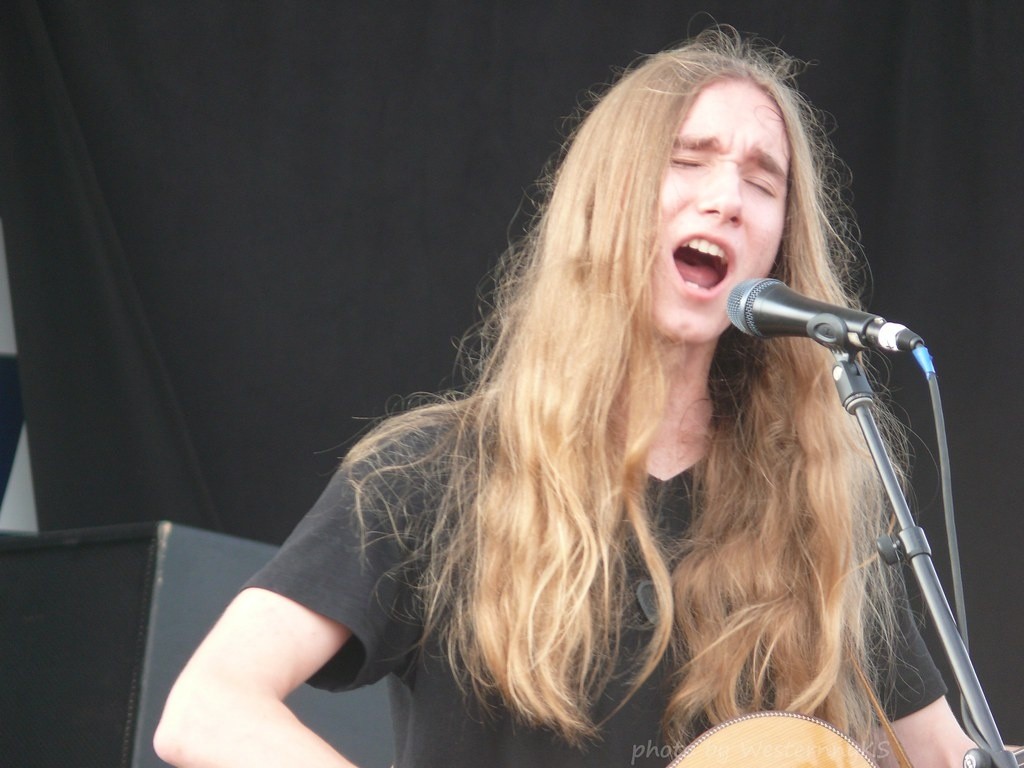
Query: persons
[[147, 42, 1024, 768]]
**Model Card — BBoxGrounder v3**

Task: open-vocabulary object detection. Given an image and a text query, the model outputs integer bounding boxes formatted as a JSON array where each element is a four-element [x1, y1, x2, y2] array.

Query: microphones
[[727, 279, 925, 352]]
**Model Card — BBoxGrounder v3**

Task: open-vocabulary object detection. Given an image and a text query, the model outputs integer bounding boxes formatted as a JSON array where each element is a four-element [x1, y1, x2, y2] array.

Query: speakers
[[1, 521, 401, 768]]
